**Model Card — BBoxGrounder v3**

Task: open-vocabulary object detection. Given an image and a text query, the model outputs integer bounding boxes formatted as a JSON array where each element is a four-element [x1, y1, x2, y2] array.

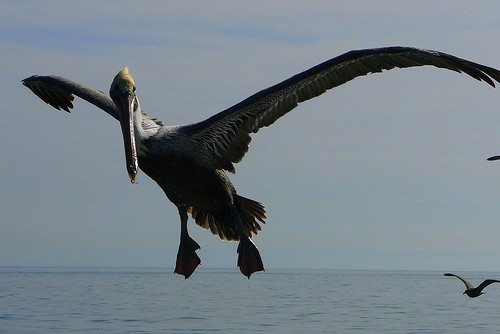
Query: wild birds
[[20, 46, 500, 281], [443, 273, 500, 298], [487, 156, 500, 161]]
